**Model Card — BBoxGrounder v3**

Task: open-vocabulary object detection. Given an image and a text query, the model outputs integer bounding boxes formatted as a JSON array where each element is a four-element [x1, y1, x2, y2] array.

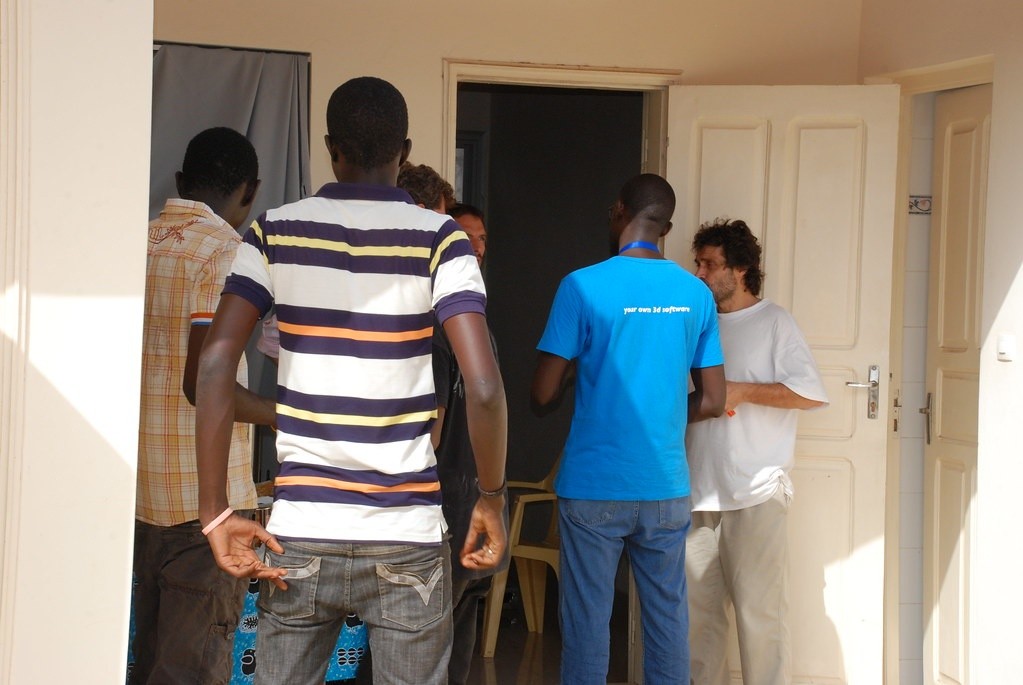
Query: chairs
[[479, 452, 567, 658]]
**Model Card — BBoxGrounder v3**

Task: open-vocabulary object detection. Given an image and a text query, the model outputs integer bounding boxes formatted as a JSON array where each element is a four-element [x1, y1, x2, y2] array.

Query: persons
[[532, 174, 726, 685], [195, 77, 508, 685], [256, 160, 499, 685], [686, 220, 829, 685], [123, 126, 263, 685]]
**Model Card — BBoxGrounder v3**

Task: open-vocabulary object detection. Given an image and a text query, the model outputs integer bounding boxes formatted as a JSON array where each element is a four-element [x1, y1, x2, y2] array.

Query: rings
[[488, 549, 493, 554]]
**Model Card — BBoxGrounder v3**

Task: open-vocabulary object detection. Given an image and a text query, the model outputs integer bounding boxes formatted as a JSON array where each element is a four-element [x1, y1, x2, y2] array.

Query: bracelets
[[474, 478, 507, 496], [201, 507, 232, 536]]
